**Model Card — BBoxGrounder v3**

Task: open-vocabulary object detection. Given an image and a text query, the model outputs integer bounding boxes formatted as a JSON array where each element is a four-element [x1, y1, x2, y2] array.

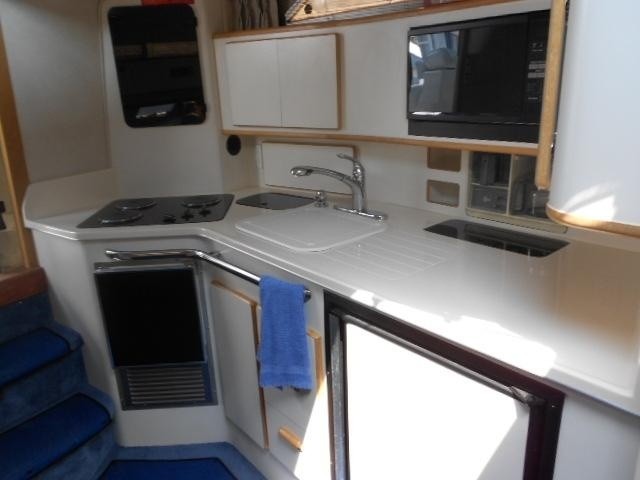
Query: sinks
[[234, 189, 317, 211]]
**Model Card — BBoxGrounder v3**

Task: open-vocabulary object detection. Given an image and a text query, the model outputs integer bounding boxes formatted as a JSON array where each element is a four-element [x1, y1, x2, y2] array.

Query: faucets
[[290, 153, 367, 211]]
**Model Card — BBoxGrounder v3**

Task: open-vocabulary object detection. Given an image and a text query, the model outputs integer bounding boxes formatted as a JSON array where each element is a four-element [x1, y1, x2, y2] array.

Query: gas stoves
[[76, 192, 235, 229]]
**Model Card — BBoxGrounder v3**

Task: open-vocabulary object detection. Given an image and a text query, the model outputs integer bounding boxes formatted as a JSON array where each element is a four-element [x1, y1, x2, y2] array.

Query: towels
[[254, 275, 314, 393]]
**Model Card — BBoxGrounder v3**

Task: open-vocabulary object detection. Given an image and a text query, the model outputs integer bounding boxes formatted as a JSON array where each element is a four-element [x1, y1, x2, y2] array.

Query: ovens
[[94, 261, 210, 369]]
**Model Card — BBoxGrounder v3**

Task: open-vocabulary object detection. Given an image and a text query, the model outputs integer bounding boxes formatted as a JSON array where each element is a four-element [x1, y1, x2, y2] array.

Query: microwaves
[[405, 11, 551, 144]]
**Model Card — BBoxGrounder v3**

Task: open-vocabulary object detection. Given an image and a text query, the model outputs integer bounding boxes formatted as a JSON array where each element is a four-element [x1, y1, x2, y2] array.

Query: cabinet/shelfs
[[202, 255, 338, 480], [212, 30, 348, 140]]
